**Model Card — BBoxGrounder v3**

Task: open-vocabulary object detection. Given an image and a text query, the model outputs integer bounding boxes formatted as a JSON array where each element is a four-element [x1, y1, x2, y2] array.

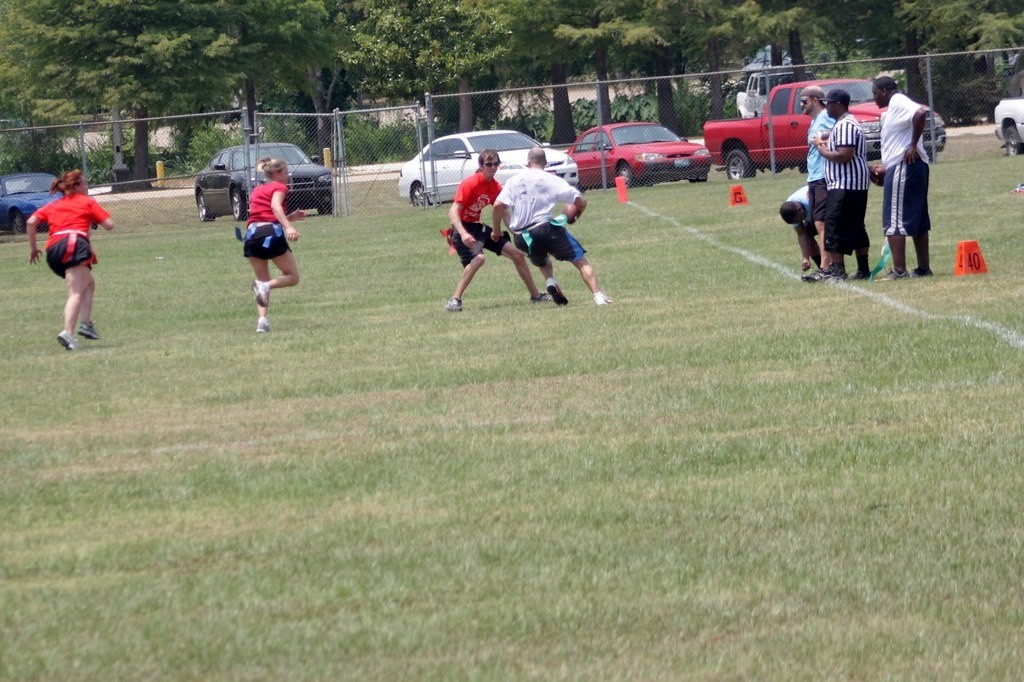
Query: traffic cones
[[953, 238, 988, 275], [729, 183, 748, 208]]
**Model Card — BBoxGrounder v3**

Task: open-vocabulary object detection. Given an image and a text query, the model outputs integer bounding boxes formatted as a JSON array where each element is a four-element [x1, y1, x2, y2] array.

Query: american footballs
[[562, 202, 577, 224], [869, 166, 886, 187]]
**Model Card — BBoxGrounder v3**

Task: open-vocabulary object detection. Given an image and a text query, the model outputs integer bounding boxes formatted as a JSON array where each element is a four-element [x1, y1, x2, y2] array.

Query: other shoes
[[851, 272, 871, 279], [909, 270, 934, 278], [825, 266, 846, 281], [882, 270, 909, 280]]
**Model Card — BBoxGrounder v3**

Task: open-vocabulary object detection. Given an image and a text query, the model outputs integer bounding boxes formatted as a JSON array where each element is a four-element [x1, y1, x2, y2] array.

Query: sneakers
[[447, 298, 463, 311], [251, 280, 270, 307], [78, 322, 101, 339], [546, 281, 569, 306], [57, 331, 78, 350], [530, 292, 551, 303], [595, 296, 613, 305], [257, 322, 269, 333], [801, 269, 832, 282]]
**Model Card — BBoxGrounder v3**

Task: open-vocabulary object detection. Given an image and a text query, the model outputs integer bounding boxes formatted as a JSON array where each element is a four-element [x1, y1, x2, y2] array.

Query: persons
[[799, 86, 871, 282], [780, 185, 872, 280], [491, 146, 613, 305], [243, 157, 308, 334], [871, 75, 934, 281], [444, 150, 552, 312], [27, 170, 115, 350]]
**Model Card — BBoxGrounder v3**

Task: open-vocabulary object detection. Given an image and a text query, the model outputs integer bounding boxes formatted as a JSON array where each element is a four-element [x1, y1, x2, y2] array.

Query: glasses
[[482, 162, 499, 167]]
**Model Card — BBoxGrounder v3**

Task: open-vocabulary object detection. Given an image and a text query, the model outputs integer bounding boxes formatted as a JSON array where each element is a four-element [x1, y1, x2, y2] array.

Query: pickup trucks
[[734, 72, 820, 119], [702, 80, 947, 180], [994, 97, 1024, 156]]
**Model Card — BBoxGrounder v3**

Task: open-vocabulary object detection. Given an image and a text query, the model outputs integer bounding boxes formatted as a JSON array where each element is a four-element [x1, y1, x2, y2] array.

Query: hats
[[819, 89, 850, 103], [800, 86, 825, 97]]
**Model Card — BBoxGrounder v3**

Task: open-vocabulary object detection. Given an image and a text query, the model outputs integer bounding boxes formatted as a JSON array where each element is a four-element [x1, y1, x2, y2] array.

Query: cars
[[398, 130, 581, 208], [566, 122, 713, 193], [0, 172, 98, 237], [194, 142, 334, 222]]
